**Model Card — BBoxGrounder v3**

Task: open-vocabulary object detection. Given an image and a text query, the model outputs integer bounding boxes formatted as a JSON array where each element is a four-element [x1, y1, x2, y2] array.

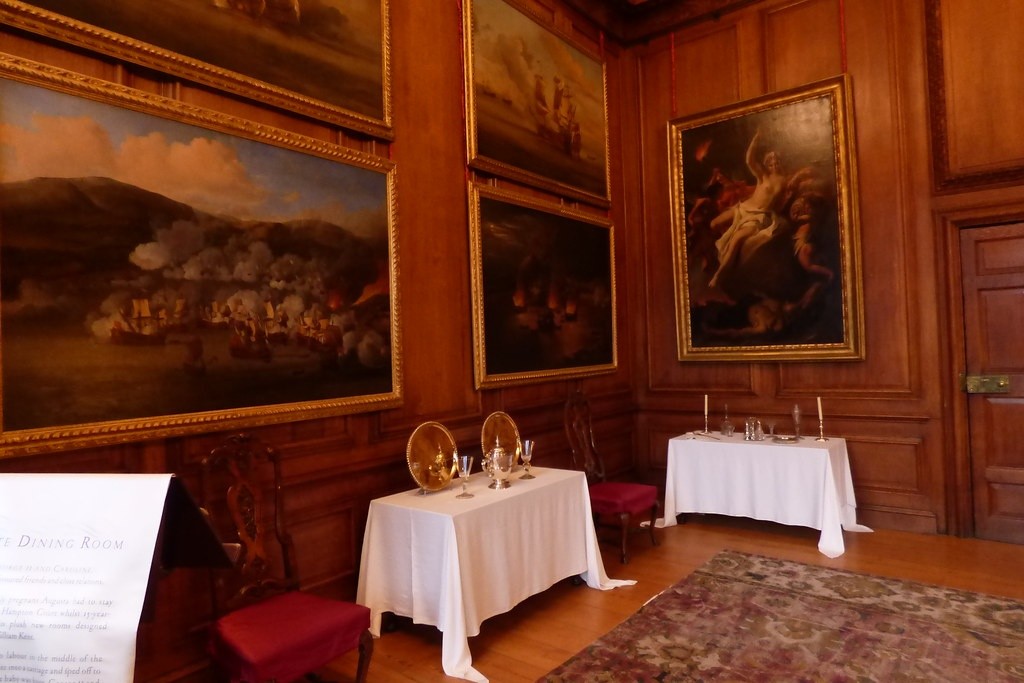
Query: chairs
[[199, 435, 374, 683], [564, 389, 660, 565]]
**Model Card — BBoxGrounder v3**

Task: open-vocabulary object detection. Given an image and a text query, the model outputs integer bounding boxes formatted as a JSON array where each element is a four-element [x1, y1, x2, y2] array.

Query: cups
[[745, 417, 764, 440]]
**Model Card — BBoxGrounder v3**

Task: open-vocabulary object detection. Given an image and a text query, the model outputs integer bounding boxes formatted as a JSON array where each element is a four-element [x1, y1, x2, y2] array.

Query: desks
[[356, 465, 637, 683], [640, 429, 875, 559]]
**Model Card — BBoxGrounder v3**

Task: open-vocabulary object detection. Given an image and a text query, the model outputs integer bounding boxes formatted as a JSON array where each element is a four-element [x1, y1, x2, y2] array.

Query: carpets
[[536, 550, 1024, 683]]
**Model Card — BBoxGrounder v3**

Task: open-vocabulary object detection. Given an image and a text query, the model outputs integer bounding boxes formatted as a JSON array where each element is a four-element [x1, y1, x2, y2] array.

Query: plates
[[407, 421, 458, 491], [772, 437, 798, 443], [480, 410, 521, 472]]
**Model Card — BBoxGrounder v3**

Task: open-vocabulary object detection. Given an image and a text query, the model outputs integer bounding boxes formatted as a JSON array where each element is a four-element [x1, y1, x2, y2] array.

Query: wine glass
[[765, 416, 778, 438], [517, 440, 536, 480], [454, 456, 475, 499], [790, 404, 806, 440], [720, 400, 734, 437], [491, 453, 513, 496]]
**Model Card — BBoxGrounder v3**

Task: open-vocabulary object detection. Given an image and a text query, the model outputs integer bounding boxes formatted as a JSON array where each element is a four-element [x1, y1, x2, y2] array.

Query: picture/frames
[[0, 50, 403, 454], [467, 180, 619, 390], [464, 0, 611, 210], [666, 73, 867, 363], [0, 0, 398, 141]]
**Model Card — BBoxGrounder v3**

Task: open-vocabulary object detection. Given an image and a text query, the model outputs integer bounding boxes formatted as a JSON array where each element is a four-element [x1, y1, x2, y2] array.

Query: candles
[[817, 397, 823, 420], [705, 394, 708, 418]]
